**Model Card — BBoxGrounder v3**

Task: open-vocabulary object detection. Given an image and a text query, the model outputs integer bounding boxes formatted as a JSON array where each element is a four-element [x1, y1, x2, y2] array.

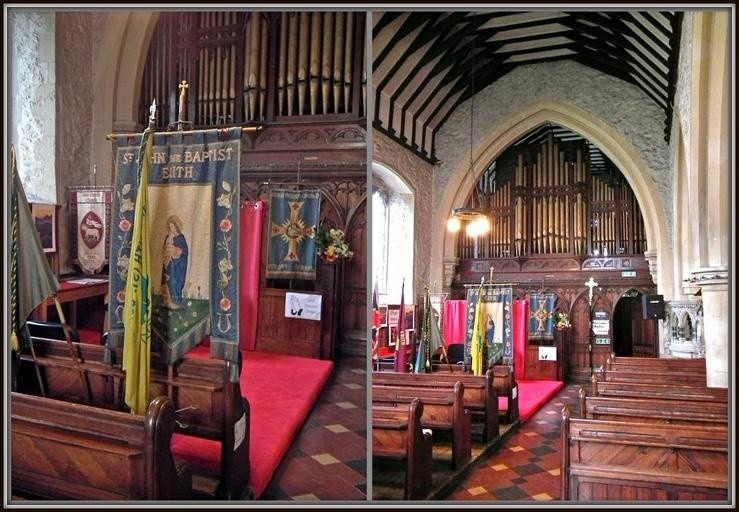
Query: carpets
[[498, 378, 565, 424], [56, 321, 335, 499]]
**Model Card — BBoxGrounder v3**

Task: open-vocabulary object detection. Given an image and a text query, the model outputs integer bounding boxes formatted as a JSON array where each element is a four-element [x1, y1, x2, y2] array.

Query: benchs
[[559, 351, 728, 500], [9, 390, 213, 501], [371, 361, 520, 500]]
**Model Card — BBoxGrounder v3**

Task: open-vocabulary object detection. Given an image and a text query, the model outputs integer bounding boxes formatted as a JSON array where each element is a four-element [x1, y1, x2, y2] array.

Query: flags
[[11, 125, 321, 412], [372, 285, 556, 375]]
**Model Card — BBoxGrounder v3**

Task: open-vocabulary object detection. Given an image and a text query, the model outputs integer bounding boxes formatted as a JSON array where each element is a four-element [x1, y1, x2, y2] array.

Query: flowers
[[557, 312, 573, 331], [311, 222, 355, 262]]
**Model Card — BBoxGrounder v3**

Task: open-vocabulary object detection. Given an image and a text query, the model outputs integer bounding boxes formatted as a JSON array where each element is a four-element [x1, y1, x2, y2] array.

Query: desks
[[378, 344, 418, 371], [26, 273, 111, 335]]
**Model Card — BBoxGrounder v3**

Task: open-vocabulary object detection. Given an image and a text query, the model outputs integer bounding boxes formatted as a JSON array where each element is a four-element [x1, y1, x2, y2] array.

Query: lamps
[[446, 12, 494, 237]]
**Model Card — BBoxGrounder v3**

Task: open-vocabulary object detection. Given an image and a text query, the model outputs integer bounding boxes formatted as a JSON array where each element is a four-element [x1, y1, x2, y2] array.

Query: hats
[[167, 215, 183, 231]]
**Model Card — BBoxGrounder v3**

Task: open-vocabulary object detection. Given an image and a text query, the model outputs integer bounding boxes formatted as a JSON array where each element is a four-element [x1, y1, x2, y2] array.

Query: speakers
[[646, 293, 667, 322]]
